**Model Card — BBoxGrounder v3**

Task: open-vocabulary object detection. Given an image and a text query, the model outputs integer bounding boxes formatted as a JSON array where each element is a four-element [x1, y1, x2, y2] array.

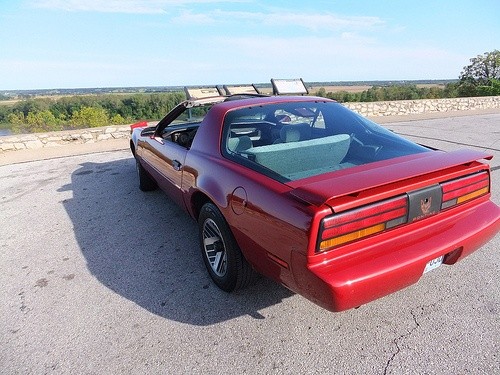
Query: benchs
[[238, 133, 350, 174]]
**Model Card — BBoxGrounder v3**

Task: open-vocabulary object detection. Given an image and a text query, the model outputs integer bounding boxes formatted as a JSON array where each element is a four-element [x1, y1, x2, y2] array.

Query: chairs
[[273, 123, 311, 144], [229, 136, 253, 157]]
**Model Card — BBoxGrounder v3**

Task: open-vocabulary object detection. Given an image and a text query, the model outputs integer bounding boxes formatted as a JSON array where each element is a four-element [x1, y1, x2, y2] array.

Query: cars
[[128, 92, 500, 312]]
